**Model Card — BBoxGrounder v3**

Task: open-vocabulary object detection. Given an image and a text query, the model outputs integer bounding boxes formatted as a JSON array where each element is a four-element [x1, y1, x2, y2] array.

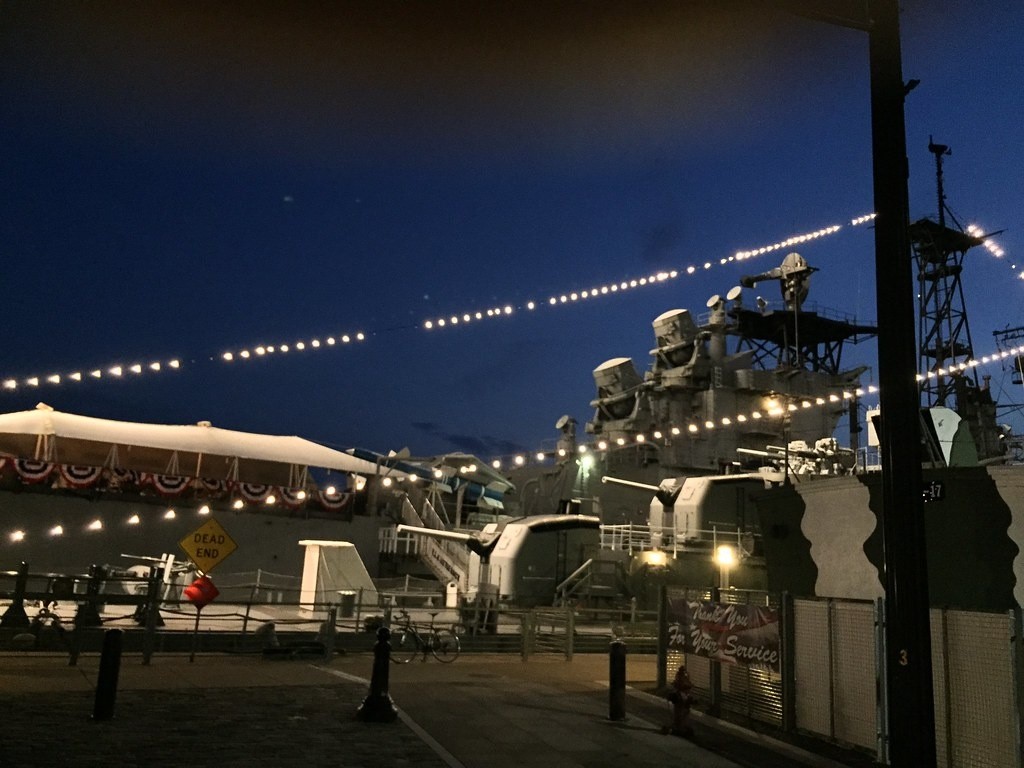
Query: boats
[[401, 252, 890, 628]]
